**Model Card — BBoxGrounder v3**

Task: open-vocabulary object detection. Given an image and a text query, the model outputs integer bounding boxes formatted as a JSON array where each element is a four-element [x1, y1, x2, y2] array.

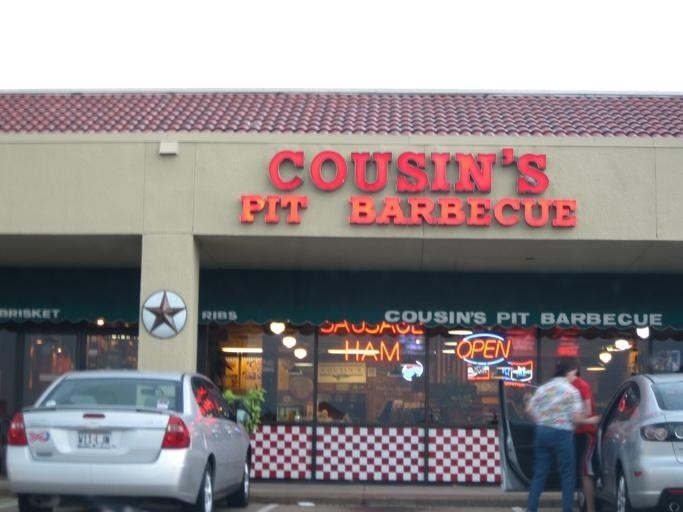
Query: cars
[[495, 368, 681, 511], [5, 368, 253, 511]]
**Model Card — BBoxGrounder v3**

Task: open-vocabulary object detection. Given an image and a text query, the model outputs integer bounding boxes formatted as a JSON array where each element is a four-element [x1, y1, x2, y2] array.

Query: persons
[[556, 352, 600, 512], [525, 355, 604, 512]]
